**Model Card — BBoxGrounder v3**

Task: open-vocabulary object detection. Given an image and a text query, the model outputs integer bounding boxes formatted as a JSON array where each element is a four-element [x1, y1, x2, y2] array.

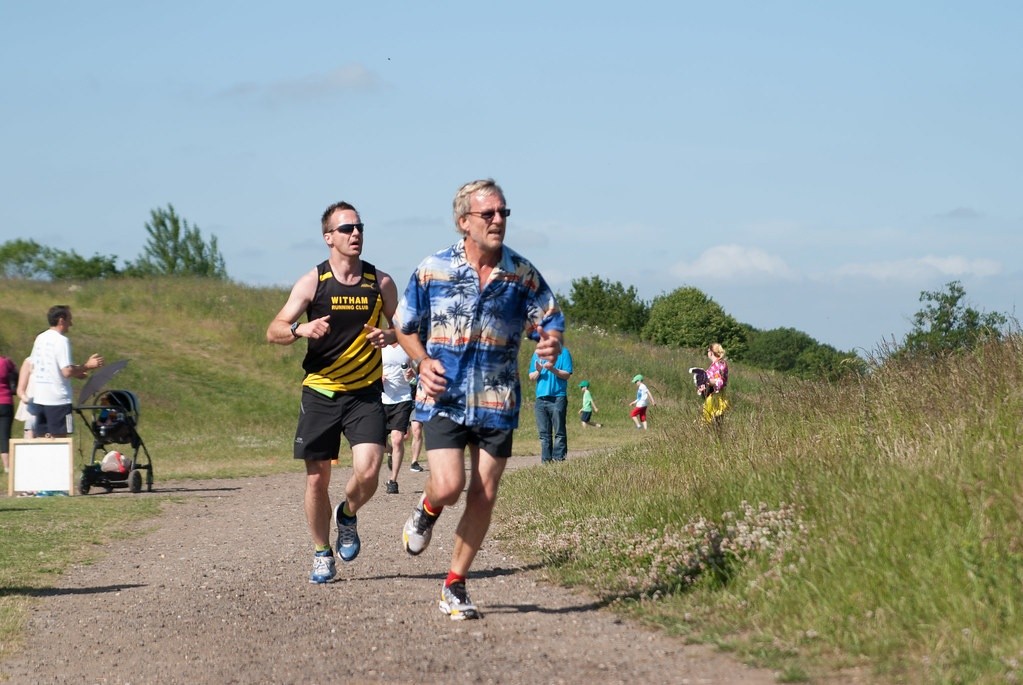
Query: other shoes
[[3, 467, 9, 473]]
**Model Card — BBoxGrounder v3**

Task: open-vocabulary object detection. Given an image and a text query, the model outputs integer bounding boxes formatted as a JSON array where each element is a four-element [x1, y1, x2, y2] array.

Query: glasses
[[328, 223, 364, 234], [705, 349, 712, 354], [463, 209, 512, 219]]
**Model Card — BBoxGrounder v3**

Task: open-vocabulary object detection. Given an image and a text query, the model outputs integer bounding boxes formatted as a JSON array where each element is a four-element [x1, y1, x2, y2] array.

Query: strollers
[[70, 388, 155, 494]]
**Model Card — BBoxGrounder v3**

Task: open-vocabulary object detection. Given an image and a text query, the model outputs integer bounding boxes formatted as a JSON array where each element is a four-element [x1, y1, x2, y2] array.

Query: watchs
[[411, 354, 429, 375], [291, 322, 302, 338]]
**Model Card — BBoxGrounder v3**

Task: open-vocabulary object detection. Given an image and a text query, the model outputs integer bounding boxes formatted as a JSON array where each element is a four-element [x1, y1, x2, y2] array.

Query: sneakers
[[410, 461, 423, 472], [439, 581, 483, 621], [385, 480, 399, 494], [309, 549, 339, 584], [387, 456, 393, 471], [401, 493, 444, 556], [334, 500, 361, 562]]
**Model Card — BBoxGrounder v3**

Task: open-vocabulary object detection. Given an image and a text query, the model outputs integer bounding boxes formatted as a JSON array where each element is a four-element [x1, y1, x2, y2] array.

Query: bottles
[[401, 363, 411, 383]]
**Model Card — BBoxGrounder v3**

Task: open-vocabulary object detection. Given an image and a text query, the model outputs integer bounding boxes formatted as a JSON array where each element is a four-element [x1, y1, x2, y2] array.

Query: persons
[[267, 202, 399, 584], [699, 343, 728, 439], [392, 179, 565, 621], [14, 306, 105, 438], [577, 380, 603, 428], [0, 356, 19, 473], [528, 345, 573, 464], [388, 400, 423, 472], [629, 374, 656, 430], [382, 342, 417, 493]]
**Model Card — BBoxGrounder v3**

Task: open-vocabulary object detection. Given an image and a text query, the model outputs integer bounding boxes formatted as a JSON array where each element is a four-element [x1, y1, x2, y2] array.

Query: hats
[[632, 374, 643, 383], [578, 380, 590, 388]]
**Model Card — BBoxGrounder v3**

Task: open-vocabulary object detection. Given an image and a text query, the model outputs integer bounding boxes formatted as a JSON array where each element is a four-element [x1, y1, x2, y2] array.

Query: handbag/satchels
[[6, 357, 19, 395]]
